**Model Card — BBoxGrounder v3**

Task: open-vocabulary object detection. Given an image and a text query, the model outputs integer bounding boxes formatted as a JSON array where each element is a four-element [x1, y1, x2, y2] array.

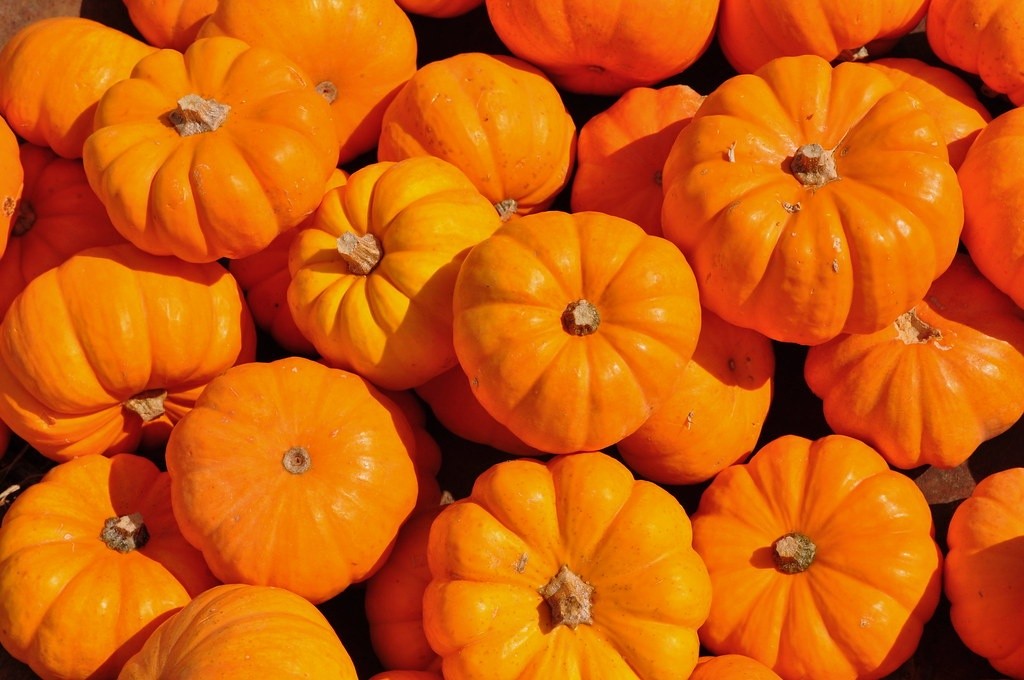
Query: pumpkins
[[1, 0, 1024, 680]]
